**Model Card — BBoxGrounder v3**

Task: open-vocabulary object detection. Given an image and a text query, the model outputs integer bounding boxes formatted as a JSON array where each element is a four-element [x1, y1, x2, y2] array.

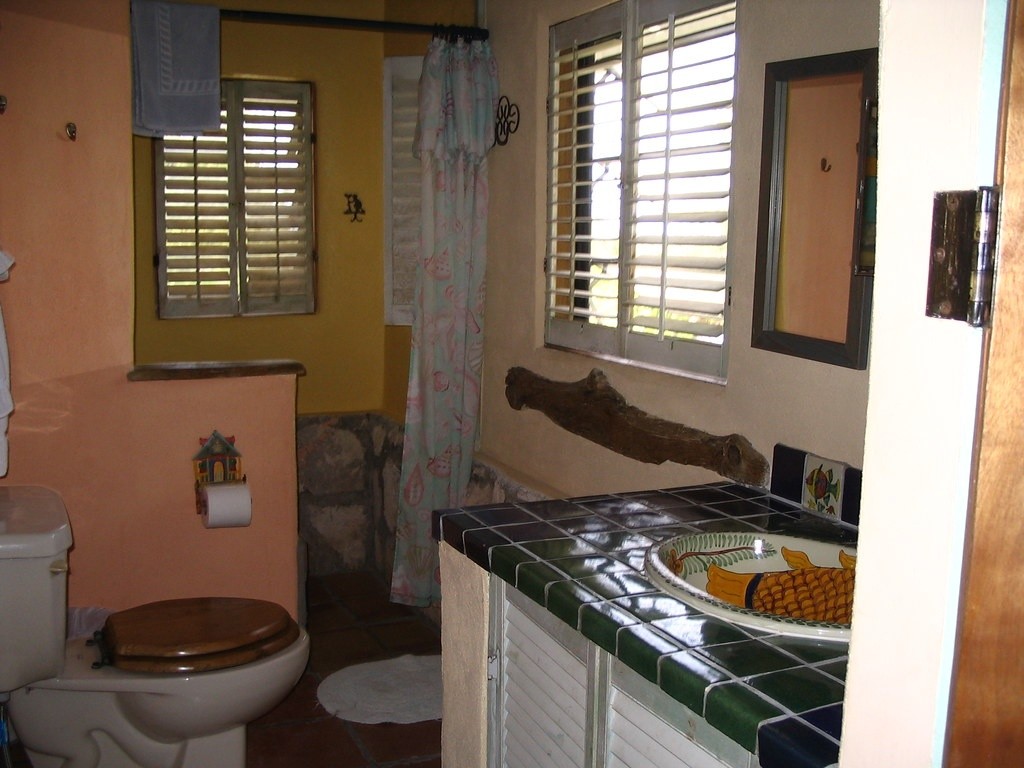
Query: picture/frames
[[750, 45, 880, 369]]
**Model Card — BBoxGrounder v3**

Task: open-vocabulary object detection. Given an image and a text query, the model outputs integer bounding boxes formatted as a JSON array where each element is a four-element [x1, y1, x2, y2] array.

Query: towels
[[131, 0, 222, 138], [0, 249, 17, 479]]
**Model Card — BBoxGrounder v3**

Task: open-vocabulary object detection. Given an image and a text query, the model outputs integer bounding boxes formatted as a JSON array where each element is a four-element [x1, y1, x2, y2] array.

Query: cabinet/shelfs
[[487, 571, 748, 768]]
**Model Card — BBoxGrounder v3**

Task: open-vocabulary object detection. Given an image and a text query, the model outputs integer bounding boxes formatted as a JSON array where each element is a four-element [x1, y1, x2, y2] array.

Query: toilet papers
[[199, 482, 252, 529]]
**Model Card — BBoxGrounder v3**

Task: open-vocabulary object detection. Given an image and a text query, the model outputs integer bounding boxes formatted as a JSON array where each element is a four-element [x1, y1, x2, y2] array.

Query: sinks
[[643, 530, 858, 644]]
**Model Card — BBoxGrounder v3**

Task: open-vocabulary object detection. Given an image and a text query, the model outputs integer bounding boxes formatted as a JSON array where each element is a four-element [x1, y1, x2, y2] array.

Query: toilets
[[0, 484, 313, 768]]
